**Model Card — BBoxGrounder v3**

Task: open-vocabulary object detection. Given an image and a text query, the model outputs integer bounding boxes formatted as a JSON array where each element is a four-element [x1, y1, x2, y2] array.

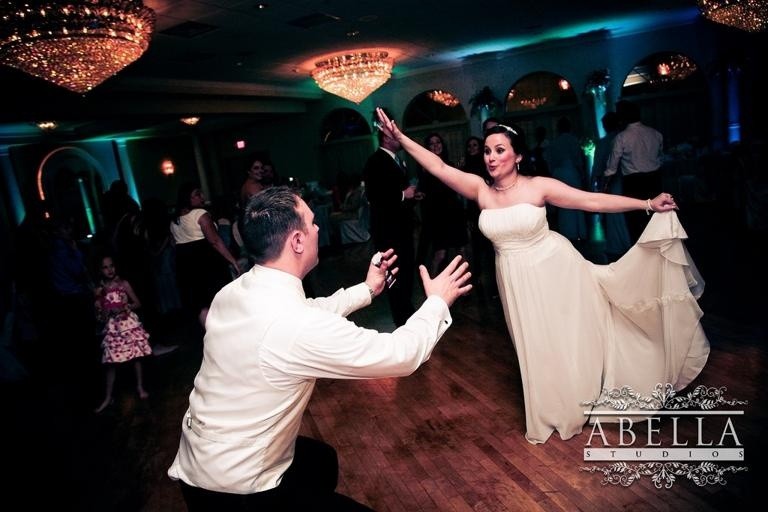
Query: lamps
[[0, 0, 156, 98], [310, 51, 393, 106], [696, 0, 768, 33]]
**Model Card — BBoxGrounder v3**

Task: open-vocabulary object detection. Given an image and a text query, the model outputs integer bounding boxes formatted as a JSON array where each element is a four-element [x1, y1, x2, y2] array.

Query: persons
[[373, 105, 710, 445], [169, 158, 306, 340], [363, 105, 670, 331], [167, 186, 475, 512], [93, 253, 153, 412], [101, 181, 141, 253]]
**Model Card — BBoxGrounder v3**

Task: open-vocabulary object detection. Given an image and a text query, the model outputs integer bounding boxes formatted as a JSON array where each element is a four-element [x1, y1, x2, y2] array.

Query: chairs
[[330, 190, 372, 247]]
